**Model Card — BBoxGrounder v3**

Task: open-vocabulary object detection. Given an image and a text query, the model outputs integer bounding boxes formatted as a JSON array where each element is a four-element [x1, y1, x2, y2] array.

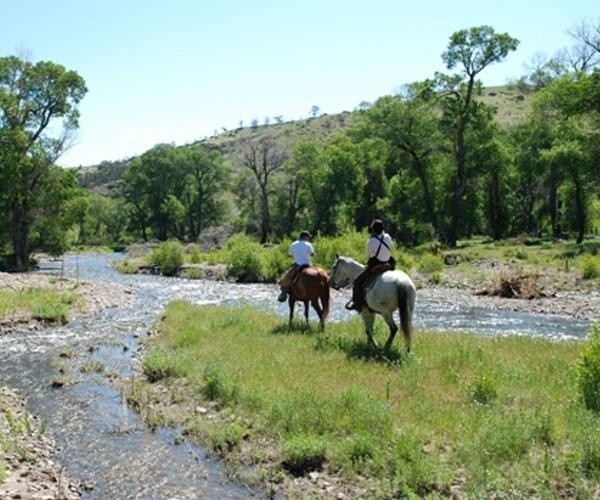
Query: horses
[[278, 267, 329, 332], [327, 252, 416, 353]]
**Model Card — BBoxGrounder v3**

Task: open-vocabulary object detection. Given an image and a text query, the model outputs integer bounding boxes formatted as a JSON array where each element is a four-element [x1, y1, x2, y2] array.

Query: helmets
[[370, 218, 384, 233], [300, 230, 313, 238]]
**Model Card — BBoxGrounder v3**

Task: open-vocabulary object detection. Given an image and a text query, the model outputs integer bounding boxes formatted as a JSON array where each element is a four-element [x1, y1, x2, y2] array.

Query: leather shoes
[[346, 303, 361, 312]]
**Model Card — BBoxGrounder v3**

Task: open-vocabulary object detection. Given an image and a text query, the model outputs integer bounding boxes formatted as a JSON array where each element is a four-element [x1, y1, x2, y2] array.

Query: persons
[[278, 231, 315, 301], [352, 219, 392, 309]]
